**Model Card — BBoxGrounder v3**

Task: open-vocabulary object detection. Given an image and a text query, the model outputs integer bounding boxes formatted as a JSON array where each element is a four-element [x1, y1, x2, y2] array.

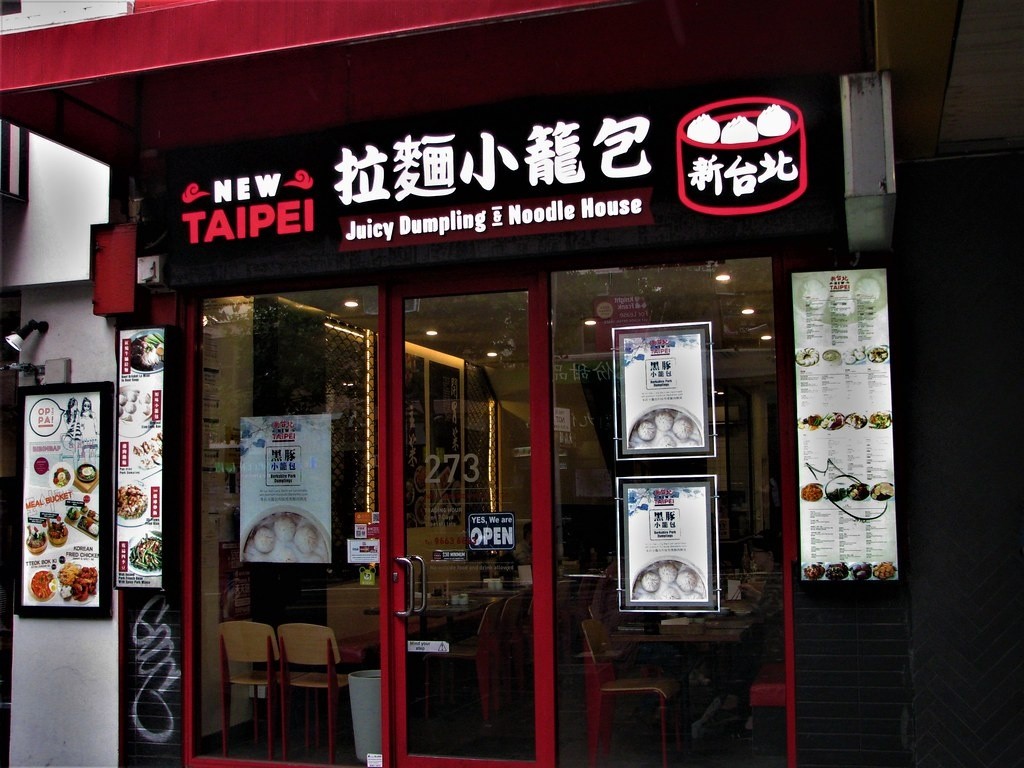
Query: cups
[[488, 581, 502, 592], [562, 560, 578, 574], [518, 565, 531, 580], [452, 595, 459, 604], [459, 594, 469, 605]]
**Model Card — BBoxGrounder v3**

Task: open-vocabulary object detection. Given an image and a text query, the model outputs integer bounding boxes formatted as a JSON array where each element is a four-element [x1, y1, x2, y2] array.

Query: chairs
[[442, 579, 574, 728], [577, 570, 789, 768], [277, 623, 349, 762], [216, 620, 310, 760]]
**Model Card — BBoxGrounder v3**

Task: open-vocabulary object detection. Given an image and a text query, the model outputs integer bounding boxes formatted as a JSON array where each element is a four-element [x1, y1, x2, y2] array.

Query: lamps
[[6, 320, 50, 353]]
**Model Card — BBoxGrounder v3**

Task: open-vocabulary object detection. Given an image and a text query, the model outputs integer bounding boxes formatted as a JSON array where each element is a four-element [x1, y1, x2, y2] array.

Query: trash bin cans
[[347, 670, 381, 761]]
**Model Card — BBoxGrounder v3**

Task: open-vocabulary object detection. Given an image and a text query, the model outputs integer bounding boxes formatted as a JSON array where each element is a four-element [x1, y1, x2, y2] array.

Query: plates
[[820, 412, 891, 429], [77, 464, 97, 483], [117, 479, 153, 527], [796, 347, 890, 367], [801, 562, 897, 580], [128, 530, 162, 576], [50, 462, 75, 491], [119, 417, 152, 438], [803, 483, 894, 501]]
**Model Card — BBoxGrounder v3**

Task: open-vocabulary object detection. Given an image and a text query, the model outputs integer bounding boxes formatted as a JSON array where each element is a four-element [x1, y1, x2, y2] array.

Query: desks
[[433, 584, 536, 721], [364, 595, 503, 716], [610, 615, 759, 744]]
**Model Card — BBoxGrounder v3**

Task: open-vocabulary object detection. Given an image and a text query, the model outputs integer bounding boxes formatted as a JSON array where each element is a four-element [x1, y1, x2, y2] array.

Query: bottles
[[742, 544, 749, 561], [733, 569, 740, 579]]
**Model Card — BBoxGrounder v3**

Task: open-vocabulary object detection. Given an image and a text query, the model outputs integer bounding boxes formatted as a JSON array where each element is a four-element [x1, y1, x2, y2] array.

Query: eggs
[[49, 579, 57, 592]]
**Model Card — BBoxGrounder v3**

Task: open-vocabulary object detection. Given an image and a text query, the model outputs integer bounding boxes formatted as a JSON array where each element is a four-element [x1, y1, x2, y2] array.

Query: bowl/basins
[[129, 331, 164, 374], [241, 505, 331, 563], [714, 607, 731, 616], [627, 405, 704, 449], [119, 386, 153, 425], [47, 526, 68, 547], [630, 557, 706, 605], [26, 533, 47, 554], [735, 611, 751, 618]]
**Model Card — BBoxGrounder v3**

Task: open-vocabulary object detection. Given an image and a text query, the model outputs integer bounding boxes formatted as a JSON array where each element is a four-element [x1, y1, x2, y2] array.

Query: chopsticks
[[730, 574, 749, 601]]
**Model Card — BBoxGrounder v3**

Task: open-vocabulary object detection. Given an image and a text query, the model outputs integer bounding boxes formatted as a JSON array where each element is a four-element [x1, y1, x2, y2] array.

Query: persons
[[592, 530, 816, 743], [513, 522, 532, 565]]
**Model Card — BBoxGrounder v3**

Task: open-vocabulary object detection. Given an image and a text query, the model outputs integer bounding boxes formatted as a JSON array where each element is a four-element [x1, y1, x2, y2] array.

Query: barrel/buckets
[[348, 670, 381, 761]]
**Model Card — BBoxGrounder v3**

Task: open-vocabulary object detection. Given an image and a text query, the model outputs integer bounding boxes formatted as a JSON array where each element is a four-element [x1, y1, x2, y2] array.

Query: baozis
[[118, 390, 152, 422], [253, 516, 325, 563], [641, 563, 704, 601], [638, 412, 699, 447]]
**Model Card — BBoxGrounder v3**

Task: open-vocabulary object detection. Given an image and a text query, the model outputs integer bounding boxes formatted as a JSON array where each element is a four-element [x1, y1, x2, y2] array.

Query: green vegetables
[[144, 332, 164, 347], [129, 536, 162, 573]]
